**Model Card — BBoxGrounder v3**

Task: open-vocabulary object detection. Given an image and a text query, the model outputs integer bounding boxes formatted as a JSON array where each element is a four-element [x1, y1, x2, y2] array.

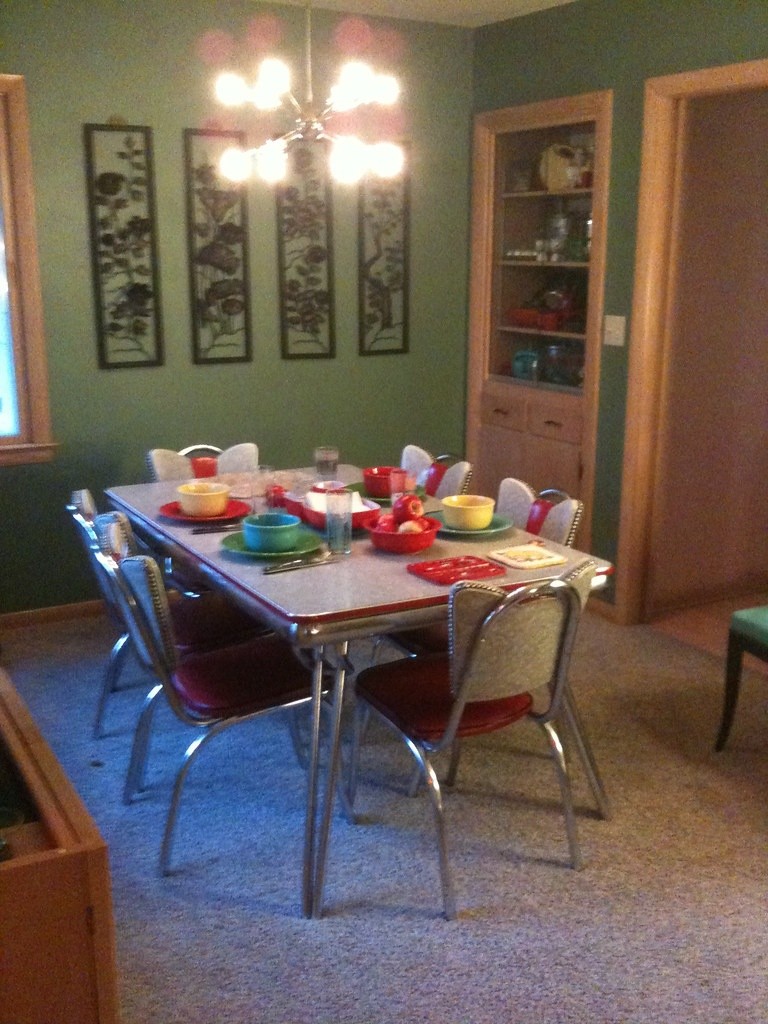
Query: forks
[[265, 551, 332, 571]]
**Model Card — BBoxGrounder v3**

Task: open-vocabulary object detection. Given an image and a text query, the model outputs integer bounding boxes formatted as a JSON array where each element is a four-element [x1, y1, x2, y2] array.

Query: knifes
[[263, 560, 337, 573]]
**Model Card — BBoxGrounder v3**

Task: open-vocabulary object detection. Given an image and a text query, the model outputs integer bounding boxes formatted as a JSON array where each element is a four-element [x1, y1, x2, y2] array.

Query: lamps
[[202, 0, 416, 185]]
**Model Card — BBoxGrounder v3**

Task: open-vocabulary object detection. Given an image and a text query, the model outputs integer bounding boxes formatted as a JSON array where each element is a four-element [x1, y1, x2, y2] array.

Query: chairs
[[147, 437, 262, 482], [69, 484, 314, 771], [342, 556, 599, 924], [364, 478, 589, 786], [712, 605, 768, 753], [94, 512, 370, 876], [399, 441, 475, 497]]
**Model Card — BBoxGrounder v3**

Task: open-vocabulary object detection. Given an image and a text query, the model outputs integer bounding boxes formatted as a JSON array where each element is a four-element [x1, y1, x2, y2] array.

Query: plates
[[193, 474, 267, 498], [220, 530, 322, 558], [313, 480, 344, 490], [422, 510, 514, 535], [159, 500, 252, 522], [343, 482, 426, 502]]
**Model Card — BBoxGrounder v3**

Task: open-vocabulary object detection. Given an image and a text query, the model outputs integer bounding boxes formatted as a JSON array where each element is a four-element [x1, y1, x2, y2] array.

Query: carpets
[[0, 590, 768, 1024]]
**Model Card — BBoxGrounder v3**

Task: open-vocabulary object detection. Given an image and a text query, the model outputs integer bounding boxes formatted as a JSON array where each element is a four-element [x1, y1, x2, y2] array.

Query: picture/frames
[[352, 137, 418, 358], [81, 120, 167, 372], [274, 131, 339, 361], [180, 123, 257, 366]]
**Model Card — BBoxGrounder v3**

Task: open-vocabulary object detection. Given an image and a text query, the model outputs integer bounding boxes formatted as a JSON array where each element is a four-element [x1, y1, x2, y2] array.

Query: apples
[[373, 494, 431, 534]]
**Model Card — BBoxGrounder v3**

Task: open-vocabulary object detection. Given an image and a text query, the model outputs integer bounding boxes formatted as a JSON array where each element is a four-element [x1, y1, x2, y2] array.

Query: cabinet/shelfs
[[456, 88, 616, 555]]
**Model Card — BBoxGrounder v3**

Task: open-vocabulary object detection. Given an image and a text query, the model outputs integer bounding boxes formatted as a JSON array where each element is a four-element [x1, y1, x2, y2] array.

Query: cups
[[325, 487, 353, 554], [250, 464, 275, 497], [315, 446, 338, 474]]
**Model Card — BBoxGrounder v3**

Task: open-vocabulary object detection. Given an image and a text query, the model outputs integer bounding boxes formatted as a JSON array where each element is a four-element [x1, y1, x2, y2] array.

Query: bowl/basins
[[442, 494, 496, 531], [363, 466, 407, 497], [362, 517, 443, 555], [300, 497, 381, 529], [283, 480, 346, 523], [241, 513, 302, 553], [177, 482, 231, 517]]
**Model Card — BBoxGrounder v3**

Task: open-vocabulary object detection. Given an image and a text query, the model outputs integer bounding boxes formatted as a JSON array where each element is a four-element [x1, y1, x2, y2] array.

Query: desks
[[105, 458, 635, 920]]
